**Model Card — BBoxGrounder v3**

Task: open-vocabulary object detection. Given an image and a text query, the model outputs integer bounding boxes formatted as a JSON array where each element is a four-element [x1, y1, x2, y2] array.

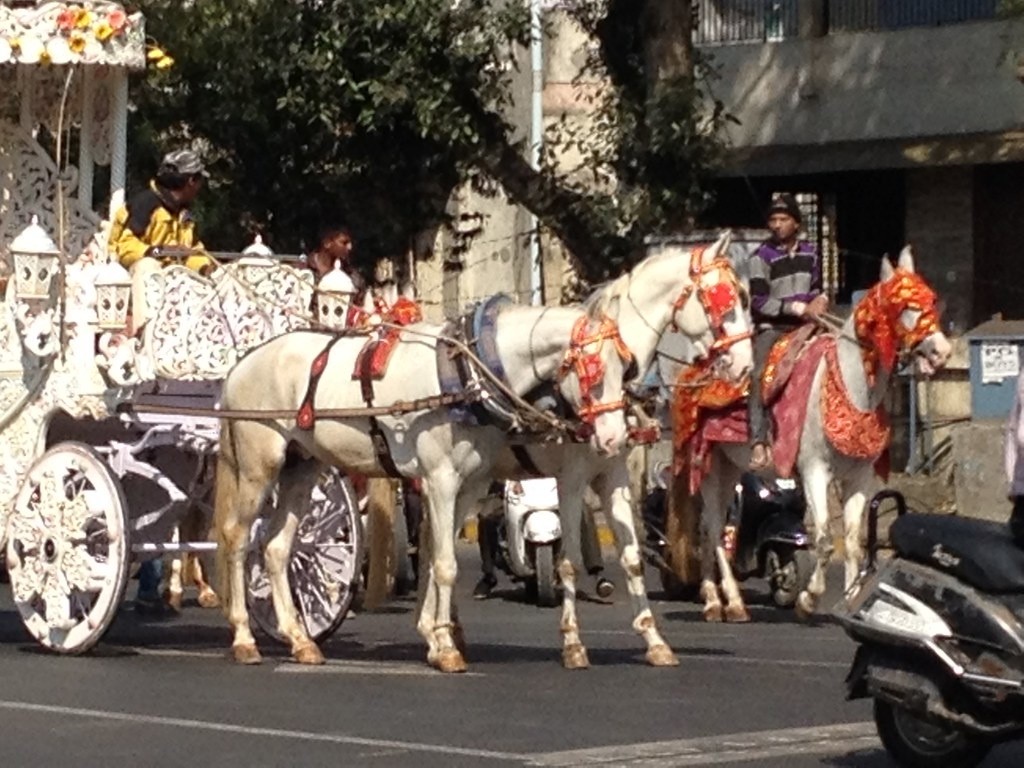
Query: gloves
[[145, 246, 164, 260]]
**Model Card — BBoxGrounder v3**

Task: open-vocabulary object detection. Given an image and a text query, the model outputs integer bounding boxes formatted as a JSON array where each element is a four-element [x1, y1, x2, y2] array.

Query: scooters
[[827, 489, 1024, 768]]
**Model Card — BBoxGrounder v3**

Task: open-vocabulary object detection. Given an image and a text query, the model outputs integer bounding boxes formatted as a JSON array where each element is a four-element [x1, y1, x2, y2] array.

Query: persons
[[746, 194, 828, 470], [107, 151, 220, 348], [474, 487, 615, 601], [1005, 369, 1024, 538], [120, 558, 178, 620], [293, 223, 365, 329], [632, 368, 672, 547]]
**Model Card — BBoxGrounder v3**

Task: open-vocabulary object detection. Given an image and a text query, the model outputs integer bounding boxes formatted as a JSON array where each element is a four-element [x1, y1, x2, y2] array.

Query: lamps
[[10, 213, 60, 304], [94, 253, 130, 328], [315, 260, 357, 328]]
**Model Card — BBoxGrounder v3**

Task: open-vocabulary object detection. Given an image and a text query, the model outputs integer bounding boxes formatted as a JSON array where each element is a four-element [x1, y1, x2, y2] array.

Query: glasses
[[772, 192, 793, 206]]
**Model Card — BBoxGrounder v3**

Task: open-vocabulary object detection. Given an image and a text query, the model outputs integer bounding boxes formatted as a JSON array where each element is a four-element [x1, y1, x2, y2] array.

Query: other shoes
[[748, 444, 769, 471], [134, 598, 175, 615], [596, 578, 615, 596], [472, 575, 497, 600]]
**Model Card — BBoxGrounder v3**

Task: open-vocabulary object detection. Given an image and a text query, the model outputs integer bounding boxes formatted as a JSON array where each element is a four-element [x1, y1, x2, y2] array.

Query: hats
[[157, 149, 211, 178]]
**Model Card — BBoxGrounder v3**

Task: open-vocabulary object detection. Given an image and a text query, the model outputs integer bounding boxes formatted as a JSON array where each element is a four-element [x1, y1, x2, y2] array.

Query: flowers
[[5, 7, 173, 70]]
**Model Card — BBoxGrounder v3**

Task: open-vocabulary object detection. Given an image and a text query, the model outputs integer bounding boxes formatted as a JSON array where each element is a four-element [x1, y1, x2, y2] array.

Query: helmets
[[767, 193, 801, 223]]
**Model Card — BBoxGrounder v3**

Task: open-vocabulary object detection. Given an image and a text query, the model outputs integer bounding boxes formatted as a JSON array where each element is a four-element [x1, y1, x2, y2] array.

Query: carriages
[[0, 1, 951, 673]]
